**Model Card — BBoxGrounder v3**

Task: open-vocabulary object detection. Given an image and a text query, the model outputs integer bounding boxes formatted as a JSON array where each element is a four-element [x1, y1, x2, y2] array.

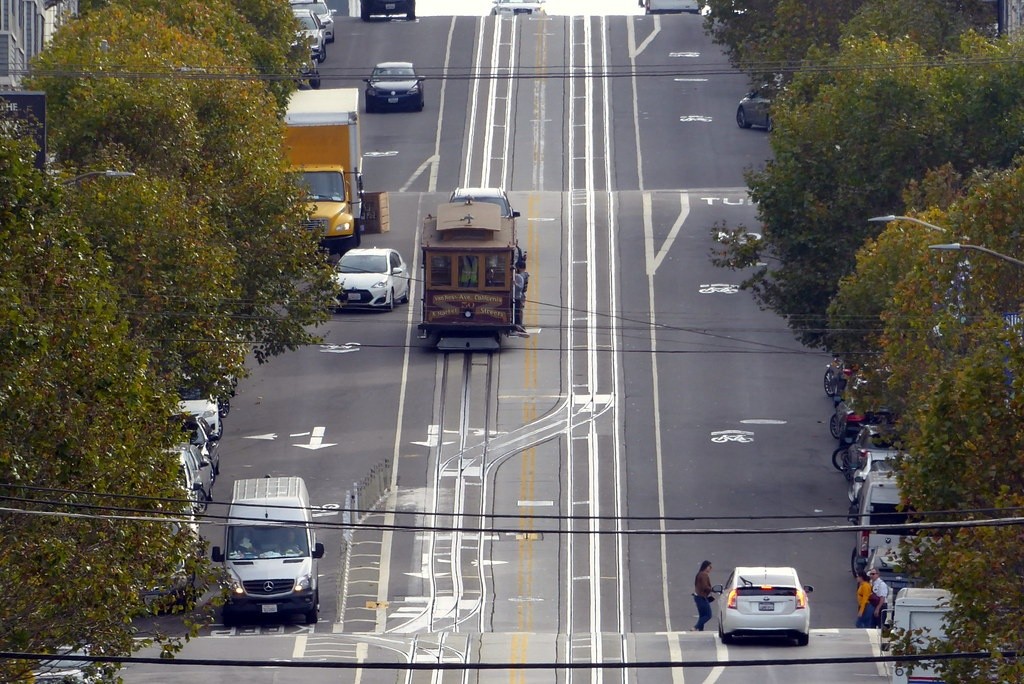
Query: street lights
[[59, 170, 136, 187], [868, 215, 946, 233], [929, 243, 1024, 267]]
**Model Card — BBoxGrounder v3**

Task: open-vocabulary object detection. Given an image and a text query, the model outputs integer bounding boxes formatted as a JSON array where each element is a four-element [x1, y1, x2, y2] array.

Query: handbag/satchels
[[868, 594, 879, 606], [707, 595, 715, 603]]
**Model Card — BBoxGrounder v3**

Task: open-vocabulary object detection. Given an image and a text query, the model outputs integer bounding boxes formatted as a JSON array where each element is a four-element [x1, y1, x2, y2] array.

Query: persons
[[460, 256, 478, 287], [690, 560, 712, 631], [516, 261, 529, 326], [856, 568, 887, 628]]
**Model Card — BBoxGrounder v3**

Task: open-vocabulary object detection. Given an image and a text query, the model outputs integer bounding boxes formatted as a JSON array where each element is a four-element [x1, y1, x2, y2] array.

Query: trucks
[[282, 87, 364, 254]]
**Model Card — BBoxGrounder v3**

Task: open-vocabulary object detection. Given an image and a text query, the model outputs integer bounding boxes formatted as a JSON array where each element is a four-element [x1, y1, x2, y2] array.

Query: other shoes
[[690, 625, 697, 631]]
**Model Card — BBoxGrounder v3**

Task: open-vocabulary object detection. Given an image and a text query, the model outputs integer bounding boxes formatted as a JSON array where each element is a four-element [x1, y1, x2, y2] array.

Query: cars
[[329, 246, 411, 312], [363, 62, 426, 113], [711, 566, 814, 647], [291, 9, 327, 63], [639, 0, 699, 14], [736, 84, 774, 133], [361, 0, 416, 21], [824, 359, 930, 603], [492, 0, 546, 16], [138, 374, 230, 610], [289, 0, 337, 43]]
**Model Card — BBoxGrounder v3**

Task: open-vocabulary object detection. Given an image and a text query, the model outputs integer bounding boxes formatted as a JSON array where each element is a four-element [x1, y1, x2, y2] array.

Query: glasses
[[870, 573, 878, 576]]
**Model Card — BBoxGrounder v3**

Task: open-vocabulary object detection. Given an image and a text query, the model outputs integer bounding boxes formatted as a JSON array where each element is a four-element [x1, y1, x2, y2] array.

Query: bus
[[417, 194, 517, 351]]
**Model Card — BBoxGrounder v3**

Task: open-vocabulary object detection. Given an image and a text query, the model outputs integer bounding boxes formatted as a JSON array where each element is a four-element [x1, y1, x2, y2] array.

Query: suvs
[[449, 187, 521, 219]]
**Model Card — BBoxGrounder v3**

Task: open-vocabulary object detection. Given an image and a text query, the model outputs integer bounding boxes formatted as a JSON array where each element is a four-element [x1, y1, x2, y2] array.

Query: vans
[[212, 476, 324, 625]]
